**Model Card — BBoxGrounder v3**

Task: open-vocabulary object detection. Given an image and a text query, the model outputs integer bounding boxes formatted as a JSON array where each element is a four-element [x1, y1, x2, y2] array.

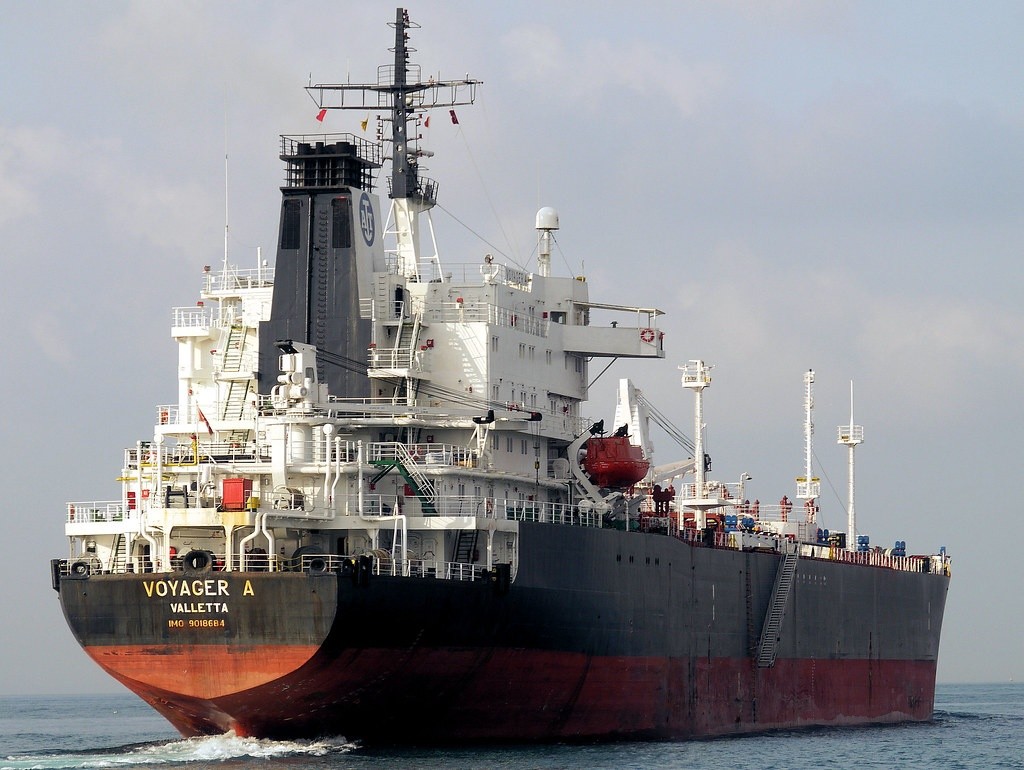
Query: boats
[[51, 8, 953, 750]]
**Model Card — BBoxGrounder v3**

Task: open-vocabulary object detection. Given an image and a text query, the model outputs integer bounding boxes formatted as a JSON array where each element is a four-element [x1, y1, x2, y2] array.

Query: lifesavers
[[70, 562, 89, 577], [486, 500, 493, 514], [309, 558, 327, 574], [640, 329, 655, 342], [161, 410, 169, 423], [183, 550, 213, 574], [408, 447, 423, 460]]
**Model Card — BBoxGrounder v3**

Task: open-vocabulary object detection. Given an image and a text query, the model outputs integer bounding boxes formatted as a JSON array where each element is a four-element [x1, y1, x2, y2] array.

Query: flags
[[316, 109, 327, 124], [425, 115, 432, 129], [197, 407, 214, 436], [359, 110, 372, 132], [448, 109, 460, 125]]
